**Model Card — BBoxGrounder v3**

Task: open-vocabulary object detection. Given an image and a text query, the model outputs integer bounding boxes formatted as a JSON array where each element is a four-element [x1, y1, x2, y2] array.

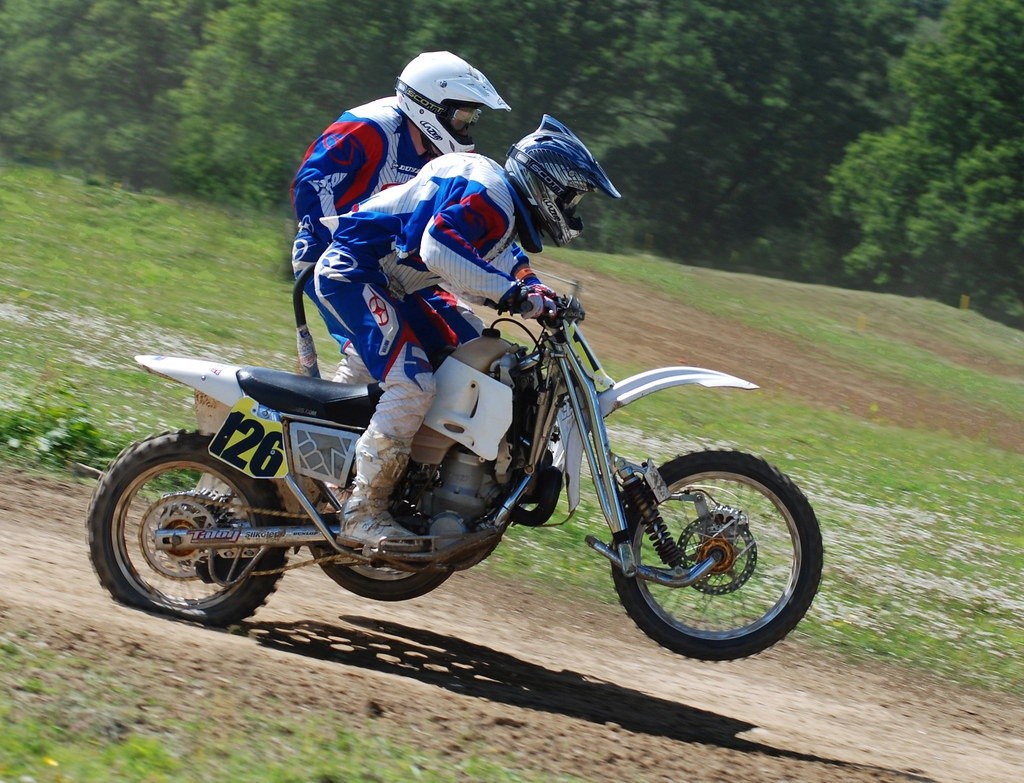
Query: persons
[[290, 49, 555, 387], [312, 115, 623, 554]]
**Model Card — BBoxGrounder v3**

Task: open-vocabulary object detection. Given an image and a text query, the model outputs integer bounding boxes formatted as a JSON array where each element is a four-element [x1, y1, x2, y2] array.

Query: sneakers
[[339, 495, 432, 548]]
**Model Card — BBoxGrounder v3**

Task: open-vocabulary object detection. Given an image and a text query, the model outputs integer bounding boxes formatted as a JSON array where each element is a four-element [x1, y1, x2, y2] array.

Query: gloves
[[521, 284, 556, 319]]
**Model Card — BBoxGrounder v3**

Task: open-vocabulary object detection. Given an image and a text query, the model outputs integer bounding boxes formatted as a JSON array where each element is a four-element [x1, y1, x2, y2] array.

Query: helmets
[[503, 113, 621, 248], [393, 51, 512, 154]]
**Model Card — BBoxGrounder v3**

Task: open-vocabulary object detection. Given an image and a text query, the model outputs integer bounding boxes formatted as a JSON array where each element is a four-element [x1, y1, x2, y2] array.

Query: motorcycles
[[86, 294, 825, 664]]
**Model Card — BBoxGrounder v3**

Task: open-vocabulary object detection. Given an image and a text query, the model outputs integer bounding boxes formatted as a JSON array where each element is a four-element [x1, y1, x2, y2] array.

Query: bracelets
[[516, 267, 534, 281]]
